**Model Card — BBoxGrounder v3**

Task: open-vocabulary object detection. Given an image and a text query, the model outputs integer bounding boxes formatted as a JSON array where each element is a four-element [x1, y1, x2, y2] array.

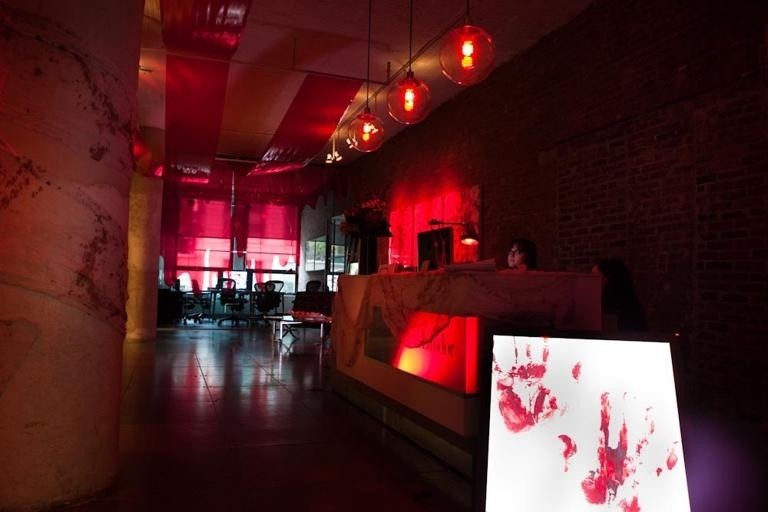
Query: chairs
[[172, 277, 286, 329], [278, 278, 337, 362]]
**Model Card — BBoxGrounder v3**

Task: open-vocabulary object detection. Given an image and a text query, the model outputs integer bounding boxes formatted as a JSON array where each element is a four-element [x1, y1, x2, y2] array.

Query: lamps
[[346, 4, 497, 152]]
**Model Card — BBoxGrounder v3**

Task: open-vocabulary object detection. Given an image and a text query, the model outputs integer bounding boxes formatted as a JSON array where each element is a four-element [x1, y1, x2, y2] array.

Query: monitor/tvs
[[417, 227, 454, 270]]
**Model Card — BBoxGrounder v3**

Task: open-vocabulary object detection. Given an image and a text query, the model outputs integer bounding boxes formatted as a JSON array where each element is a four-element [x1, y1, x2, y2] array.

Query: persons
[[504, 237, 536, 271], [591, 256, 646, 332]]
